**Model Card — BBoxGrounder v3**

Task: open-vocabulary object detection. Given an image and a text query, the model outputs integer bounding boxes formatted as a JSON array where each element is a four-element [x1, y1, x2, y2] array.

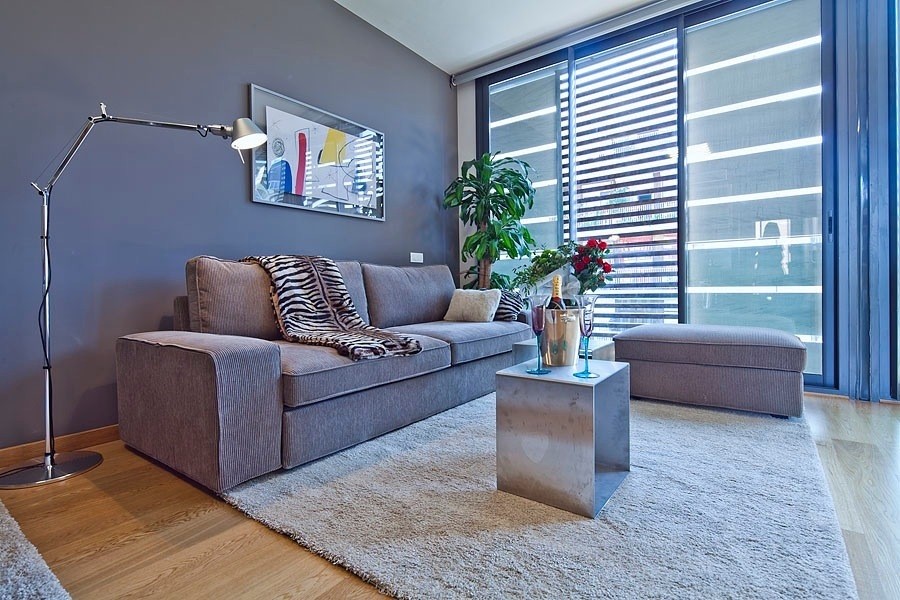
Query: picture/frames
[[250, 83, 385, 222]]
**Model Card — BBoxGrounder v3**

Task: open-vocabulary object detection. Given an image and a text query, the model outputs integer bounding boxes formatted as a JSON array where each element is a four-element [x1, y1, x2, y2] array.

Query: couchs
[[115, 265, 534, 493]]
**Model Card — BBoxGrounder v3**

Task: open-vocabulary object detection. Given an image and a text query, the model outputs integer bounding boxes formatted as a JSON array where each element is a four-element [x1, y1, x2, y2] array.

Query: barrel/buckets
[[535, 305, 586, 367]]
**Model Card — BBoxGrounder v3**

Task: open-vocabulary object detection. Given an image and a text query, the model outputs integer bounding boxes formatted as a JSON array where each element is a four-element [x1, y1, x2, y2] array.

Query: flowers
[[567, 237, 615, 306]]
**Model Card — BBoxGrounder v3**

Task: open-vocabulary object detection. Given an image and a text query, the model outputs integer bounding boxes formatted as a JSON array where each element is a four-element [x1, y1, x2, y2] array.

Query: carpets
[[219, 390, 859, 600], [0, 494, 71, 600]]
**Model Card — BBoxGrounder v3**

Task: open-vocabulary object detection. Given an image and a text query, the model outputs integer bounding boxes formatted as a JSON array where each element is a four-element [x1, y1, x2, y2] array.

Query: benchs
[[612, 324, 808, 420]]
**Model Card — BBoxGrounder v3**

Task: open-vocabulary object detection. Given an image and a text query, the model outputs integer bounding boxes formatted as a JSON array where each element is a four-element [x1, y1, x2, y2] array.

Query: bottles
[[546, 274, 566, 310]]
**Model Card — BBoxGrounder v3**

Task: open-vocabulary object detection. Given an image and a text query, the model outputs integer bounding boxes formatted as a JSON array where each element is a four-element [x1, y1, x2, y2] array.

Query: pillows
[[479, 288, 523, 320], [443, 289, 501, 322]]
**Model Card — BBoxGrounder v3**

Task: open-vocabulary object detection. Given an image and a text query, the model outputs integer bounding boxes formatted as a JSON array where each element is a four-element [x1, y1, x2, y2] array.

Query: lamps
[[0, 103, 267, 489]]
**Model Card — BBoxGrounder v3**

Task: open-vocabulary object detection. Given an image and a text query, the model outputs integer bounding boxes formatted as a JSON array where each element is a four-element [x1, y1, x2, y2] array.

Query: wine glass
[[573, 294, 600, 378], [526, 295, 552, 375]]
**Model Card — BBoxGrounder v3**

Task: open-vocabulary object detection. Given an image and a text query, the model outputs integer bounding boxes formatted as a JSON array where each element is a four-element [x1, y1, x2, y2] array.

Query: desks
[[495, 335, 630, 518]]
